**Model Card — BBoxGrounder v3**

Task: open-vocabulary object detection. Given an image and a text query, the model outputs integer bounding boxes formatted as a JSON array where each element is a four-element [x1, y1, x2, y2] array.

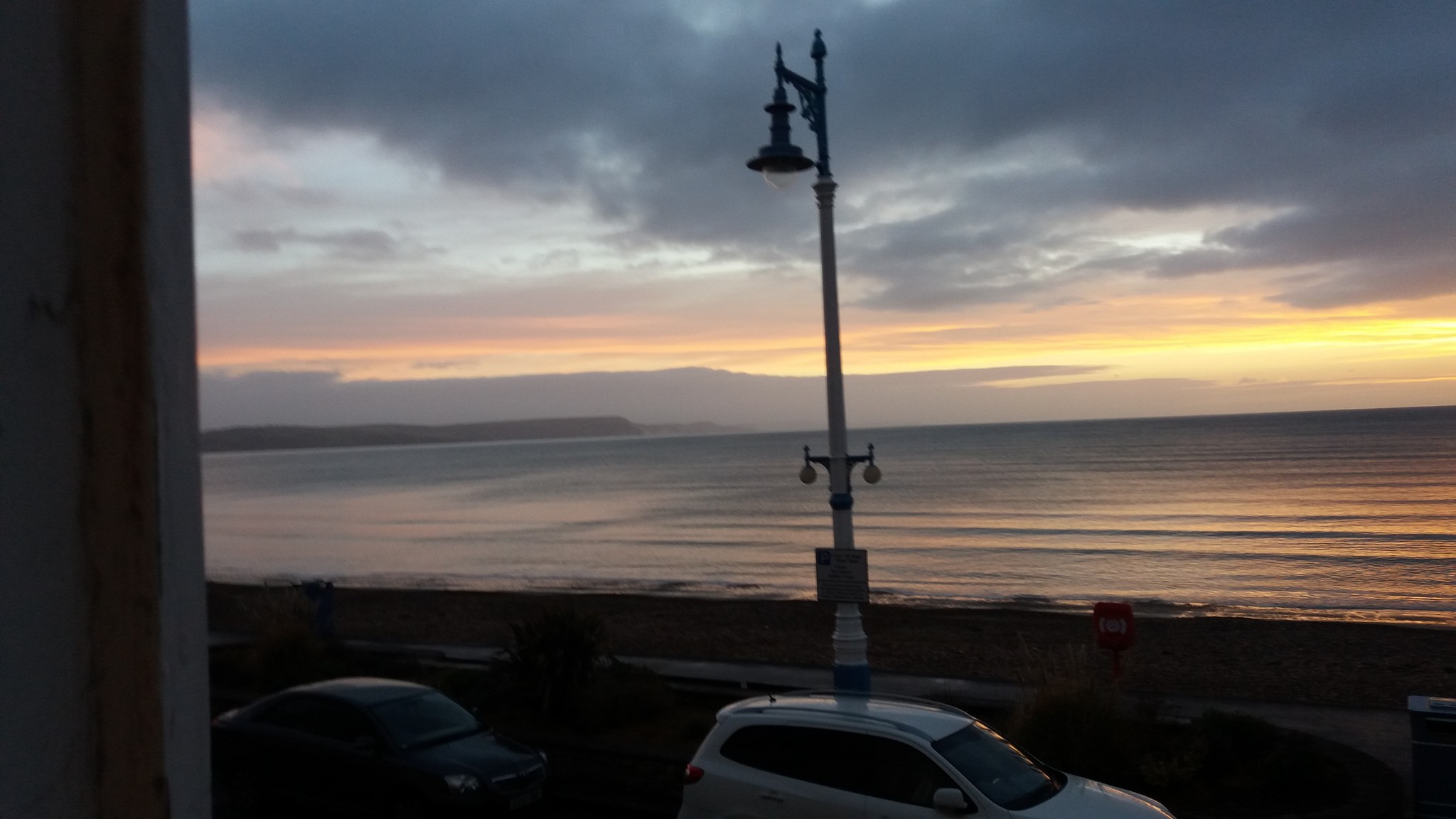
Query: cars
[[211, 676, 551, 818]]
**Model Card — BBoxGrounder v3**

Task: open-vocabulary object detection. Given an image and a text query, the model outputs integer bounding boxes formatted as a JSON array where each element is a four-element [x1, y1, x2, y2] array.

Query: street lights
[[745, 37, 884, 697]]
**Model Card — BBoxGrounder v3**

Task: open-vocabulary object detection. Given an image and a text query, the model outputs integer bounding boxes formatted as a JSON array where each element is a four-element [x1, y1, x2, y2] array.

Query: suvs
[[685, 693, 1176, 819]]
